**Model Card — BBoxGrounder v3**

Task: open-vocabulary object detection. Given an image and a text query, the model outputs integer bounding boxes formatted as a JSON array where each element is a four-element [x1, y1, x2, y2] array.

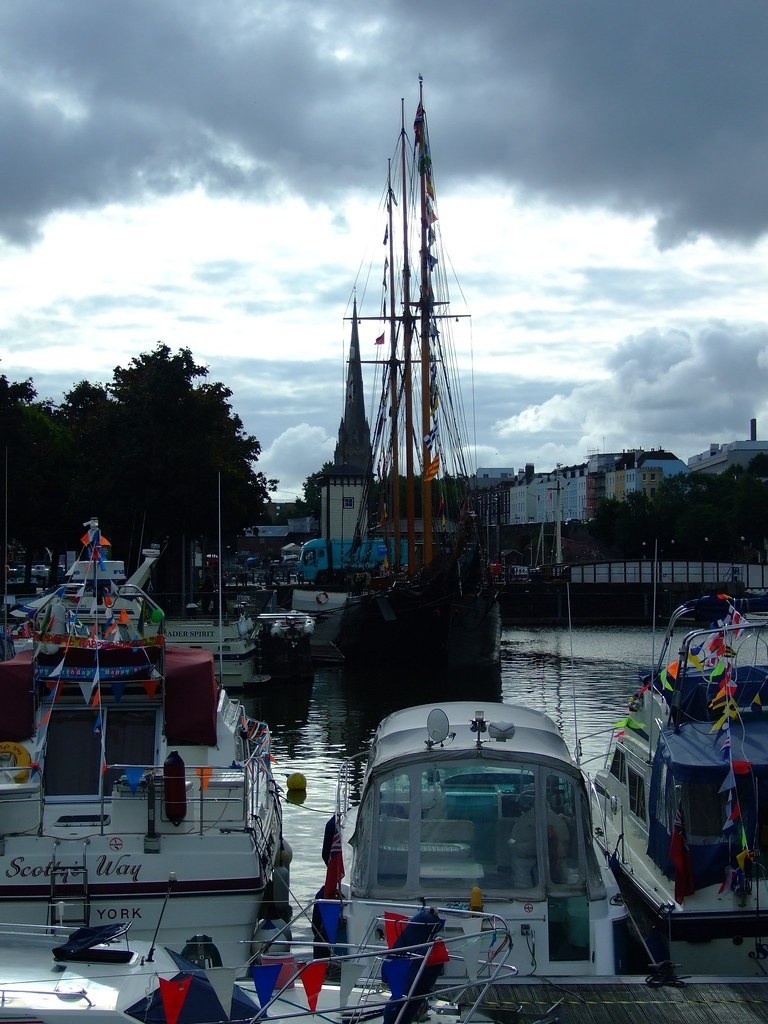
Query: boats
[[578, 538, 768, 980], [7, 557, 276, 698], [2, 517, 284, 931], [1, 898, 518, 1024], [309, 705, 629, 982]]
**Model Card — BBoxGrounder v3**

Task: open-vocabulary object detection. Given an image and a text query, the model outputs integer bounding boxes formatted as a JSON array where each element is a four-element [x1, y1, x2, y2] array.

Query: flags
[[385, 502, 392, 573], [413, 101, 438, 424], [439, 488, 445, 528], [375, 334, 384, 345], [424, 425, 440, 449], [383, 187, 397, 324], [708, 594, 749, 719], [423, 454, 440, 481], [324, 828, 345, 899]]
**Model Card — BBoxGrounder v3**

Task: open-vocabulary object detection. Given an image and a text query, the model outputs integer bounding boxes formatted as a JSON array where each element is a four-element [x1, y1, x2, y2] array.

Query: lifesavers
[[1, 742, 34, 783], [315, 591, 331, 606]]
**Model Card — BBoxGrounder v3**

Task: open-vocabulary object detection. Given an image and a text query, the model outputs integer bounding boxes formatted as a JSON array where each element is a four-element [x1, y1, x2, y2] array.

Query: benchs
[[380, 818, 475, 858]]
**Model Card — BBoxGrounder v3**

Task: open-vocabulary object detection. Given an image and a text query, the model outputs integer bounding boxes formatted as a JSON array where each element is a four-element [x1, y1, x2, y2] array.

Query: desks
[[420, 862, 484, 879]]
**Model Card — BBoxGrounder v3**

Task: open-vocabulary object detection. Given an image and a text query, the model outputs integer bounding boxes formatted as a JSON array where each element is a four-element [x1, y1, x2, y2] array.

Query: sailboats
[[256, 72, 510, 706]]
